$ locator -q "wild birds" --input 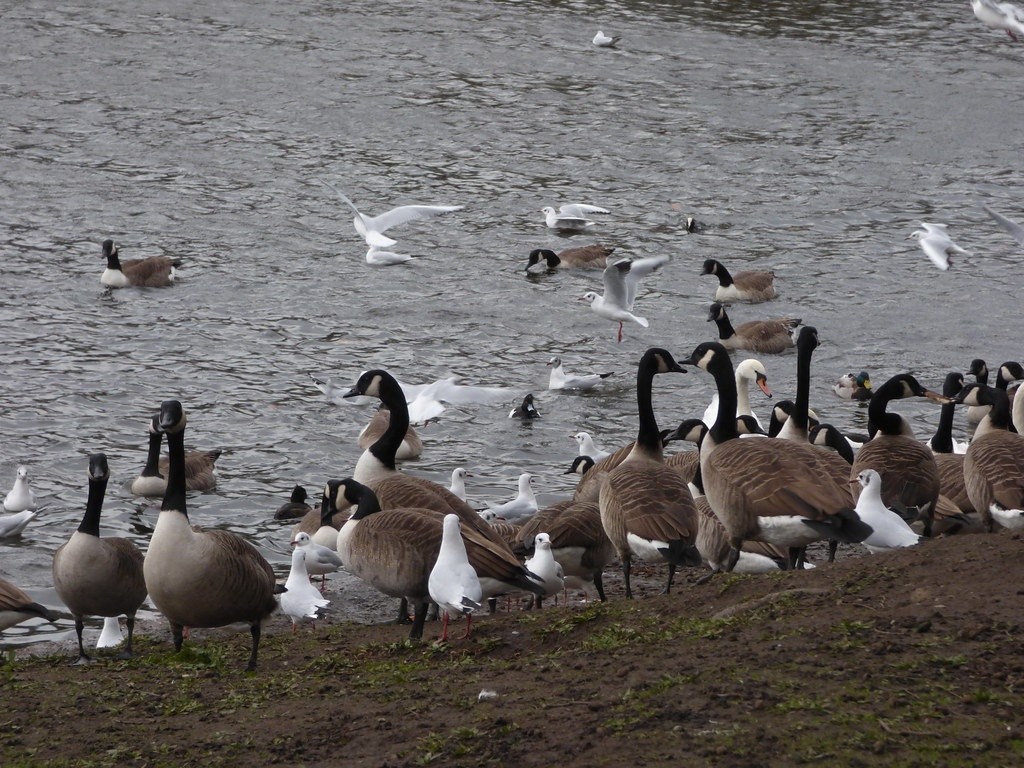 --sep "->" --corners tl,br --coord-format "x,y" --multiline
686,218 -> 707,234
982,203 -> 1024,247
315,176 -> 468,265
3,466 -> 39,511
95,614 -> 125,646
538,205 -> 611,230
577,254 -> 669,328
525,244 -> 616,274
700,259 -> 780,304
705,303 -> 806,352
0,577 -> 62,637
131,408 -> 222,495
904,222 -> 974,271
356,369 -> 524,428
545,358 -> 615,391
142,400 -> 287,678
272,325 -> 1024,646
0,502 -> 51,541
99,238 -> 182,290
51,450 -> 146,665
